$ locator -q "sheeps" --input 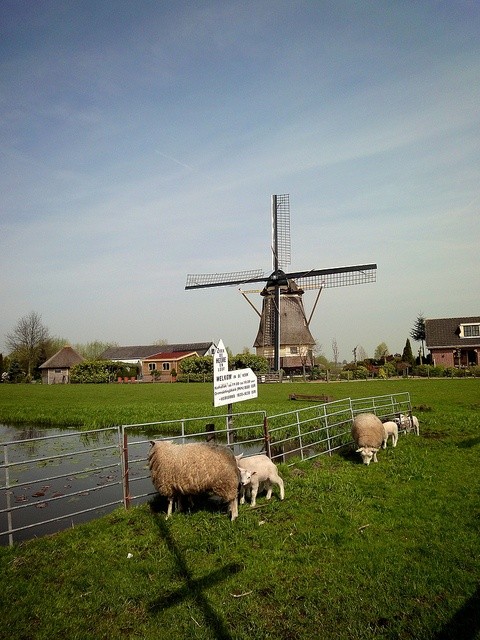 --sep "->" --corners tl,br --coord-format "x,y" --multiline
395,414 -> 421,436
382,420 -> 400,450
234,454 -> 271,498
148,440 -> 243,522
351,413 -> 386,466
238,459 -> 285,508
